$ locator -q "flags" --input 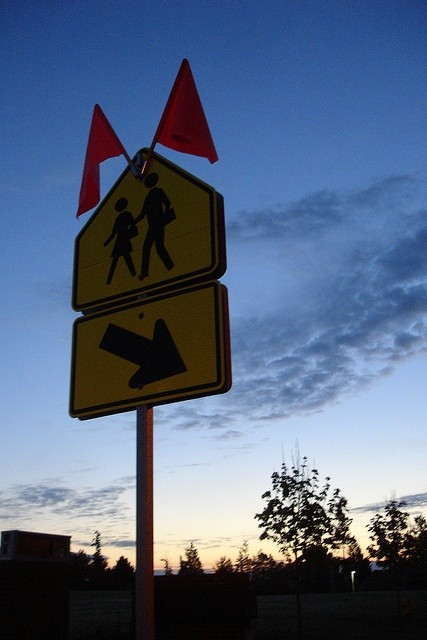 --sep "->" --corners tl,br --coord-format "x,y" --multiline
154,57 -> 218,165
74,102 -> 124,220
